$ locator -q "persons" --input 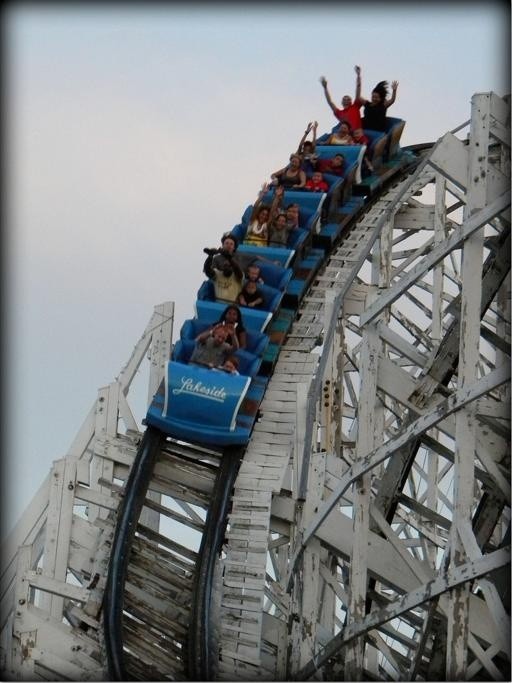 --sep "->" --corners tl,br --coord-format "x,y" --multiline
318,121 -> 352,145
309,152 -> 344,177
238,280 -> 265,308
203,246 -> 246,304
221,231 -> 281,277
208,355 -> 239,375
242,180 -> 286,248
286,203 -> 299,226
356,78 -> 400,132
187,321 -> 239,369
303,170 -> 328,193
296,120 -> 318,168
352,127 -> 374,174
318,65 -> 362,134
272,152 -> 306,189
268,202 -> 300,250
245,266 -> 263,284
206,303 -> 247,351
